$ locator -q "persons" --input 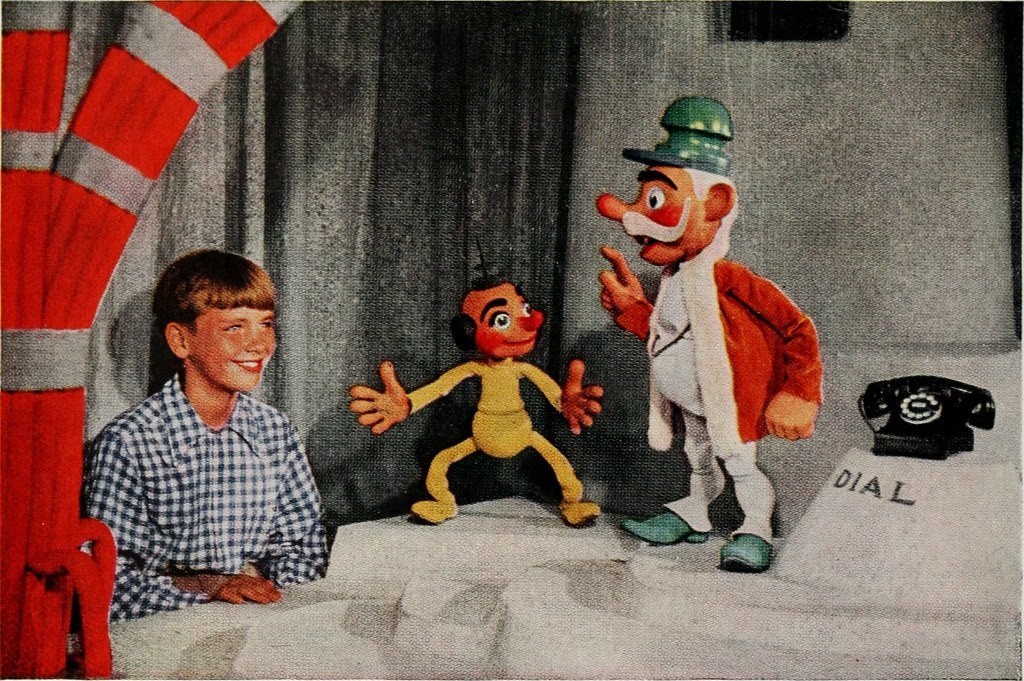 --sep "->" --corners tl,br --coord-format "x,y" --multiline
82,249 -> 328,622
595,95 -> 824,571
350,277 -> 603,528
